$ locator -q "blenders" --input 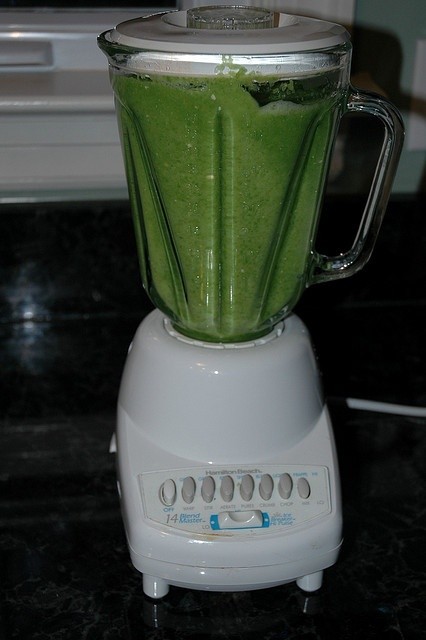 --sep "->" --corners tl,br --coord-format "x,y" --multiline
95,7 -> 408,599
